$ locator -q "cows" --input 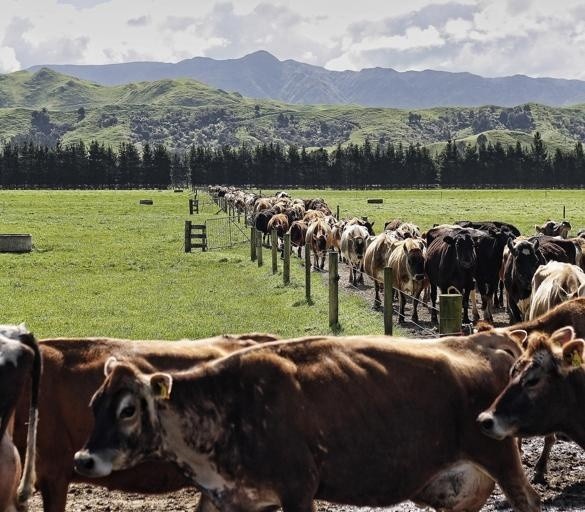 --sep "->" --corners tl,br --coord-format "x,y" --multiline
208,183 -> 376,290
475,298 -> 585,483
30,333 -> 278,512
71,333 -> 543,512
1,325 -> 41,512
364,219 -> 585,321
